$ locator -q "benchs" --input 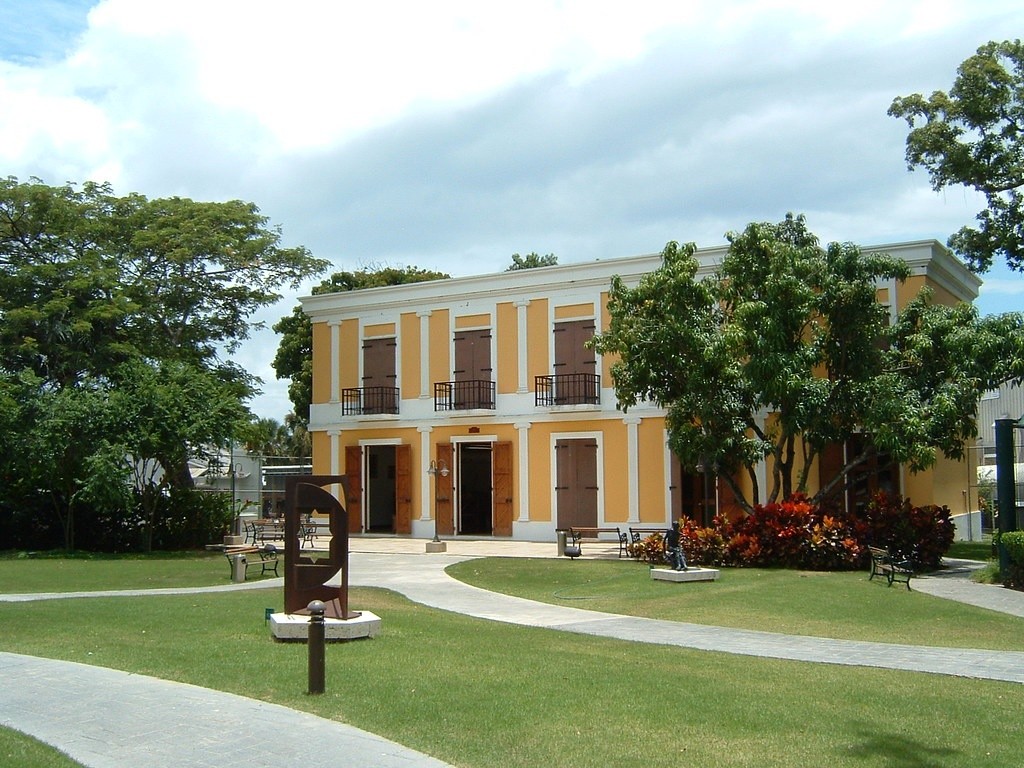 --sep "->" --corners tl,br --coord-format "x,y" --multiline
868,544 -> 914,592
243,517 -> 319,548
223,545 -> 279,581
629,527 -> 670,558
569,526 -> 629,558
